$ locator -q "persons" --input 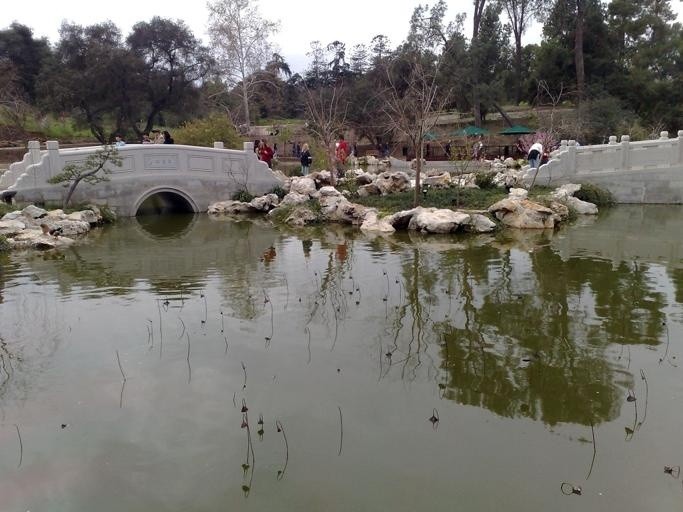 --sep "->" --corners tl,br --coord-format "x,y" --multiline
529,140 -> 543,167
292,142 -> 313,175
142,128 -> 174,144
254,137 -> 278,169
114,135 -> 125,146
